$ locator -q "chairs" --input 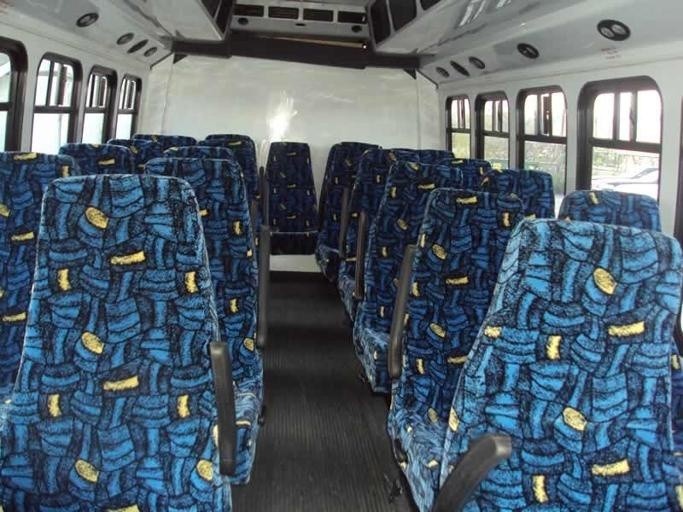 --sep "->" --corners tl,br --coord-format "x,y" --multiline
255,139 -> 318,256
315,141 -> 683,511
1,129 -> 265,512
